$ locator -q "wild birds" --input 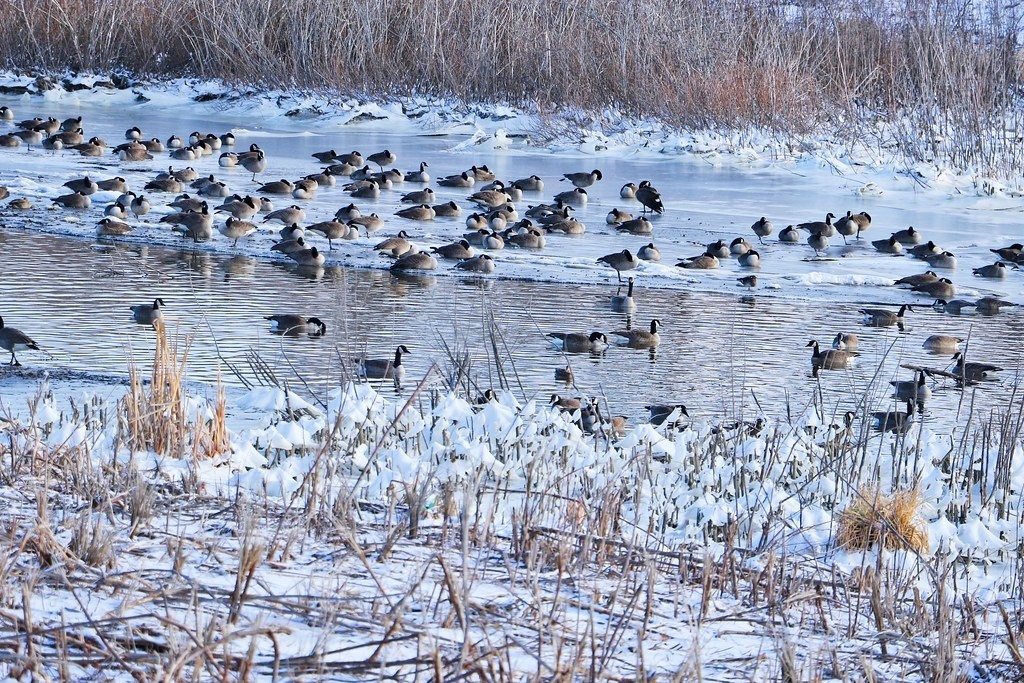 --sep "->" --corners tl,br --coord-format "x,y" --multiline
0,107 -> 1023,440
